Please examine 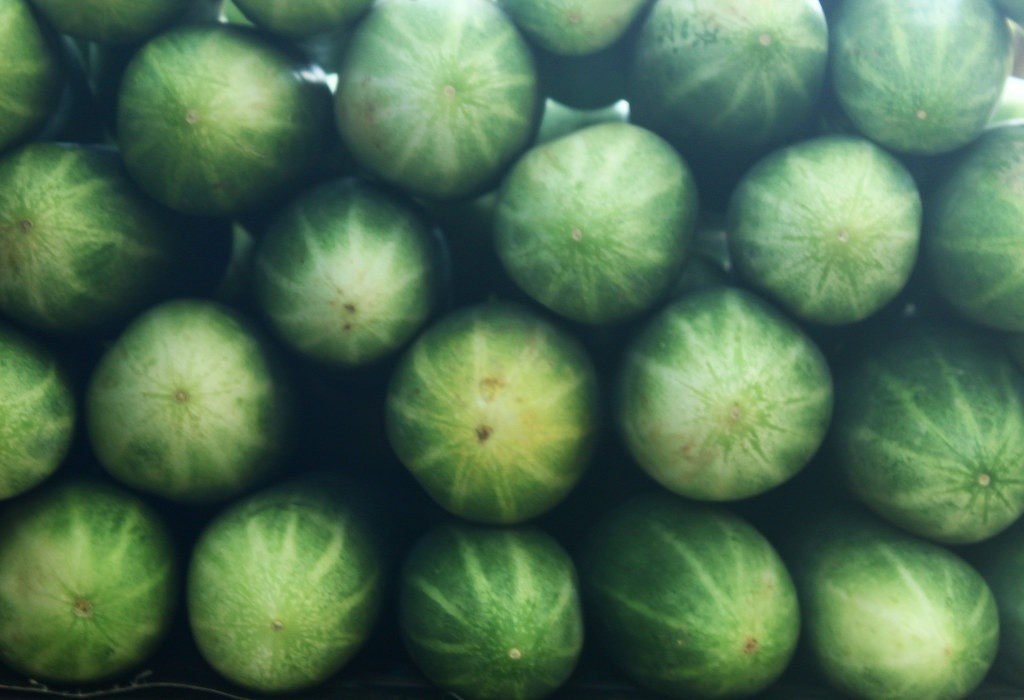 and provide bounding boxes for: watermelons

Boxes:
[0,0,1024,700]
